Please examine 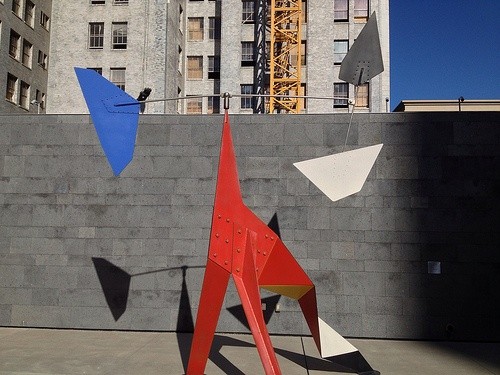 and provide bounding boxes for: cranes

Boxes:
[262,0,302,113]
